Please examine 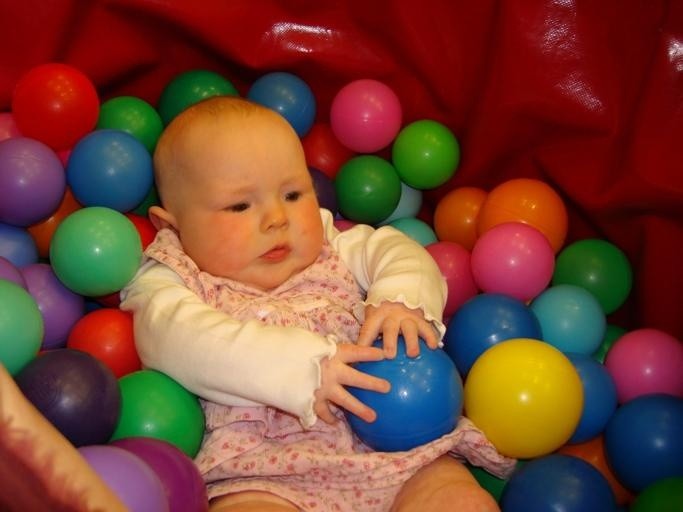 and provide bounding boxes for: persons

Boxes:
[120,96,502,512]
